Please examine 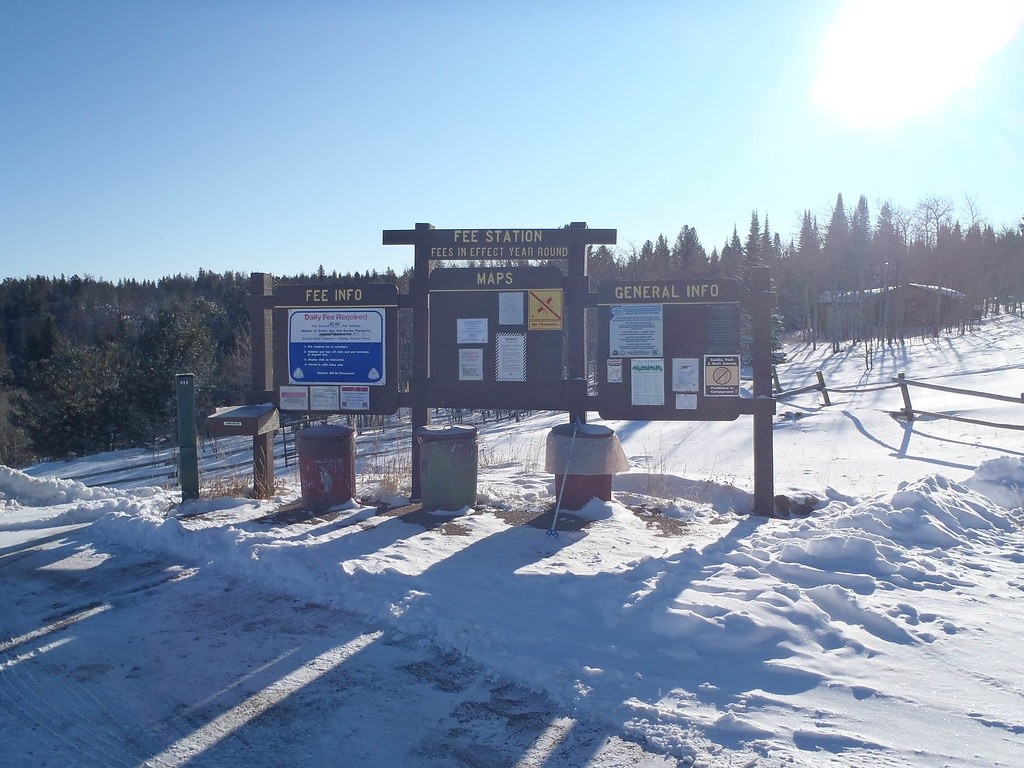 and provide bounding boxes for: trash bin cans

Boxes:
[414,423,478,515]
[294,420,356,512]
[551,423,616,510]
